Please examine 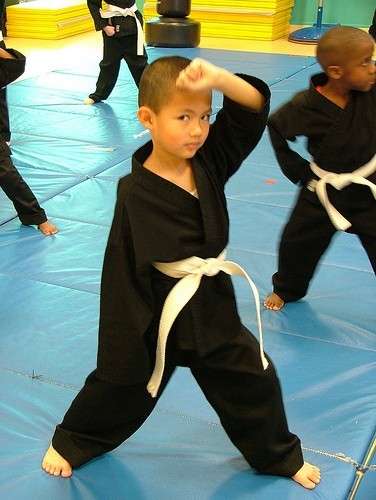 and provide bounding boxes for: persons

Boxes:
[263,26,376,310]
[84,0,149,104]
[41,56,321,490]
[0,0,58,236]
